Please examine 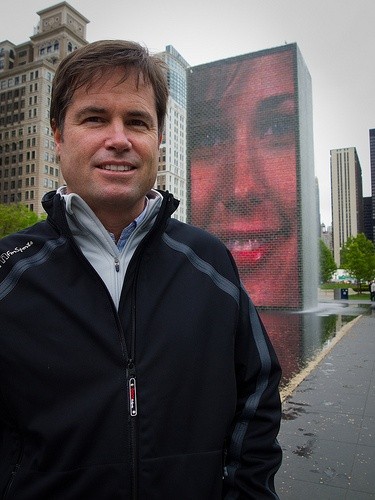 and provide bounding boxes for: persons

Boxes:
[0,40,282,500]
[369,280,375,301]
[187,52,299,309]
